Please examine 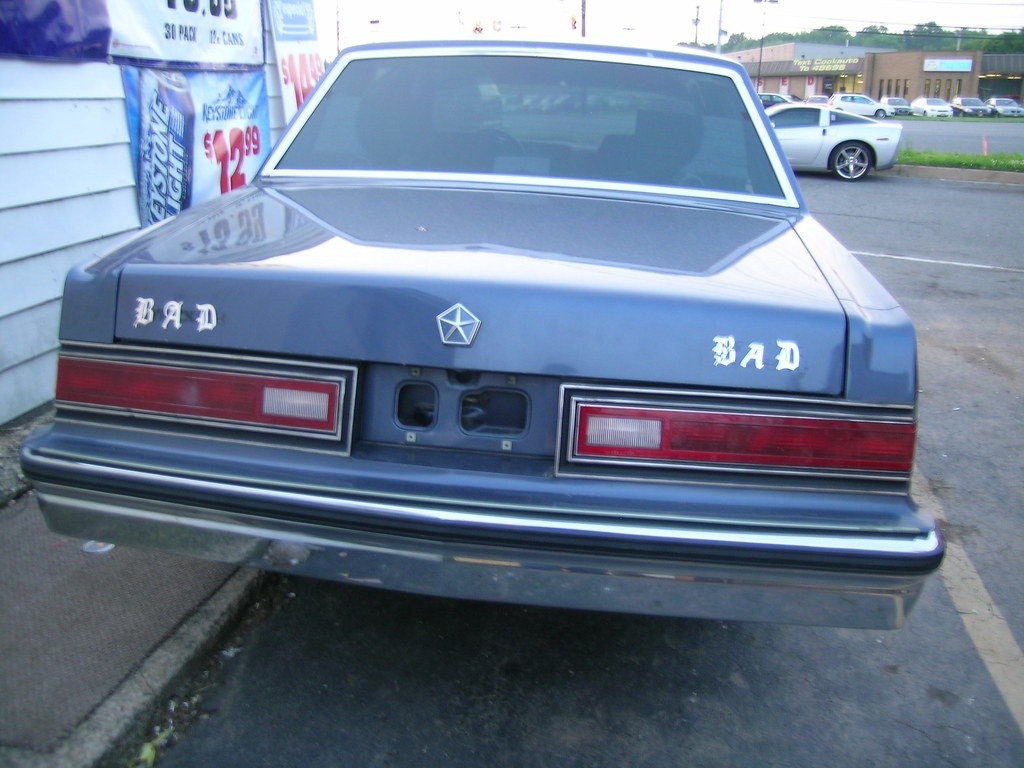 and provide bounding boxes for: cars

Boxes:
[762,101,905,184]
[948,95,993,120]
[984,97,1024,119]
[879,95,915,118]
[828,93,897,119]
[14,40,948,632]
[760,90,829,121]
[911,96,954,120]
